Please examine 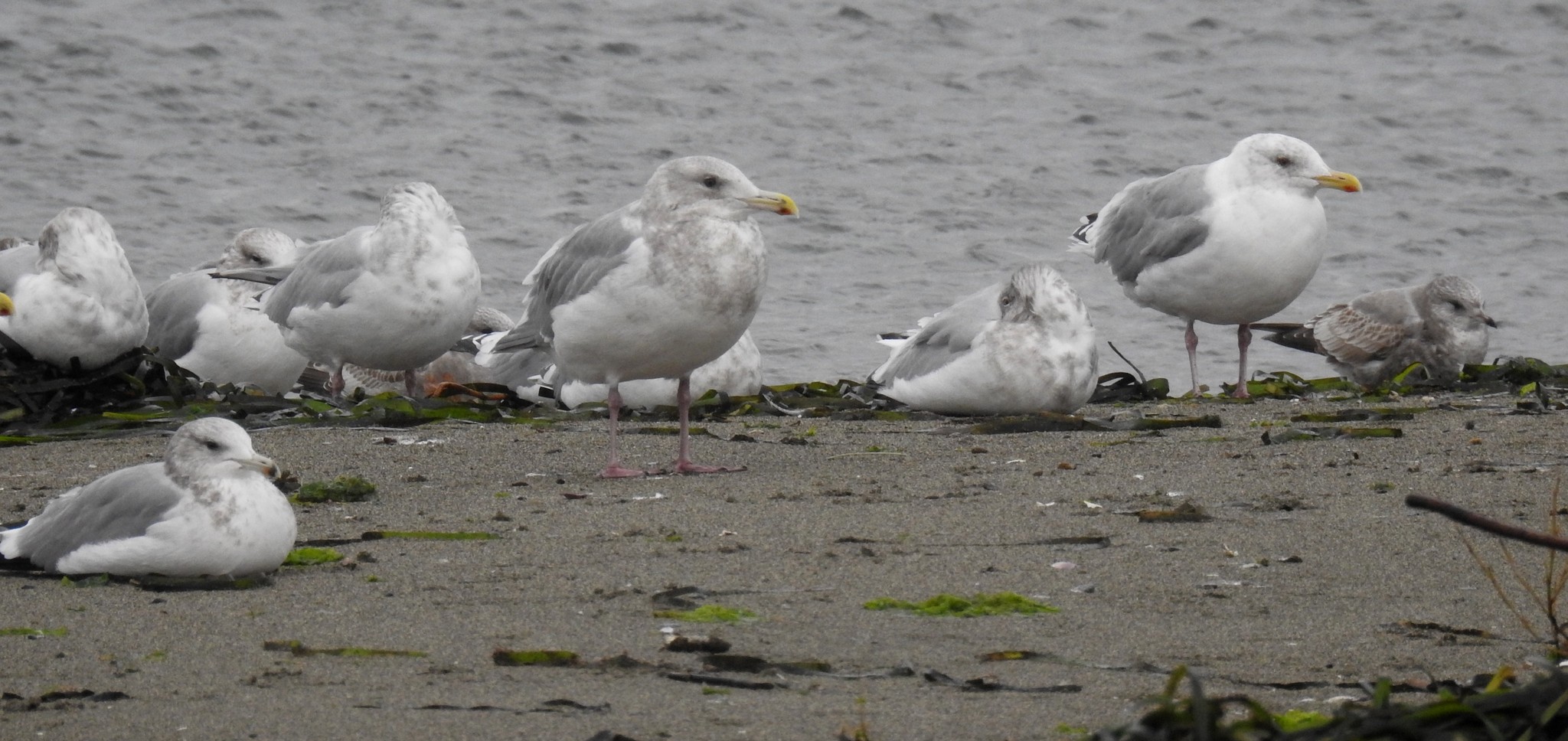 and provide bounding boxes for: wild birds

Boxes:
[850,261,1101,415]
[1064,132,1363,398]
[1251,275,1502,388]
[1,155,802,408]
[0,417,300,579]
